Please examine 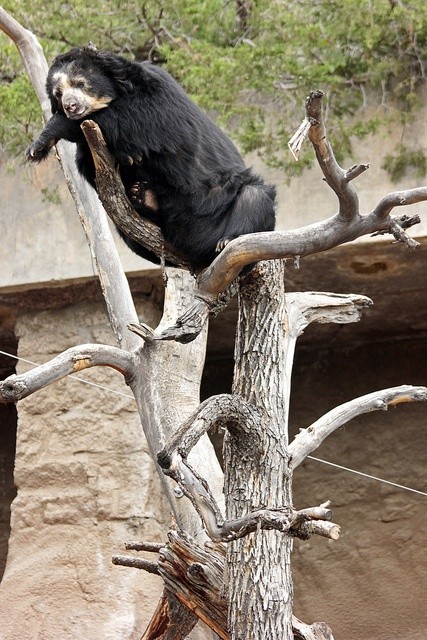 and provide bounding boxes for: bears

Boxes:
[25,46,281,275]
